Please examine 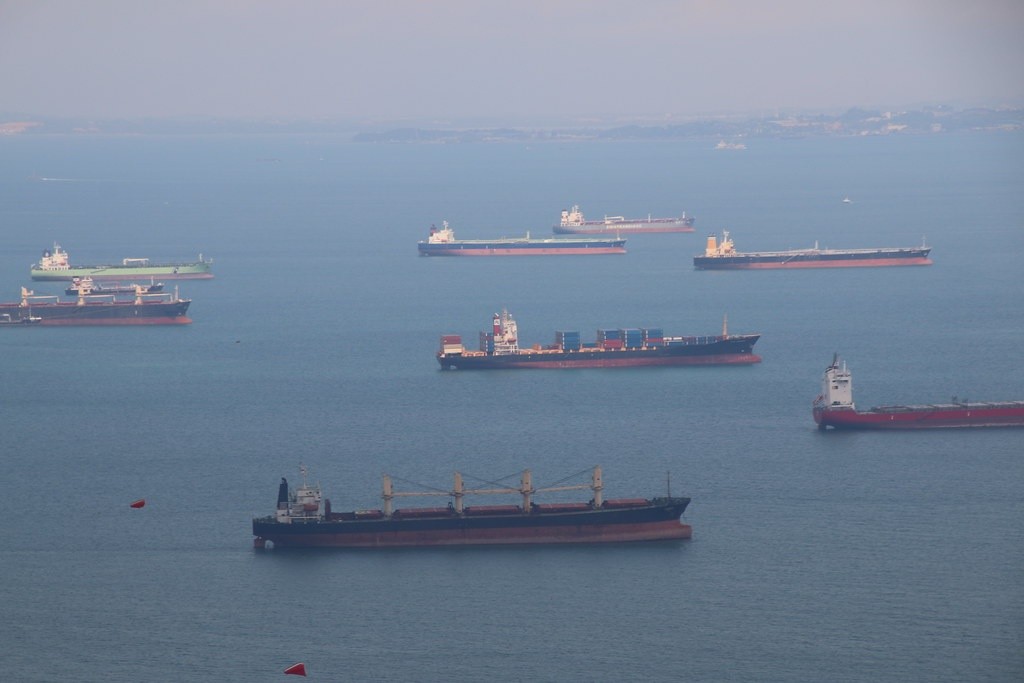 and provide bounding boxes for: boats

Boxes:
[812,360,1024,427]
[0,287,194,325]
[554,204,694,232]
[250,464,694,549]
[418,224,627,258]
[693,230,933,268]
[437,312,763,368]
[65,275,163,292]
[30,242,214,280]
